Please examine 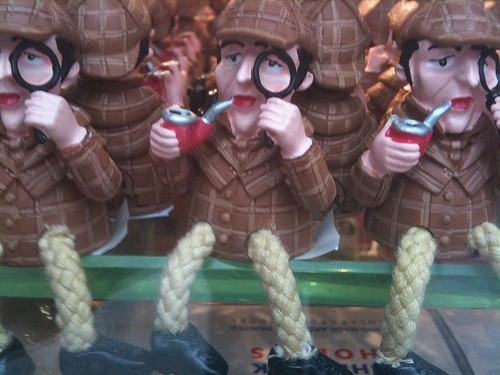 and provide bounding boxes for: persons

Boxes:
[346,0,500,375]
[299,1,419,195]
[0,0,155,375]
[150,0,355,375]
[79,1,202,213]
